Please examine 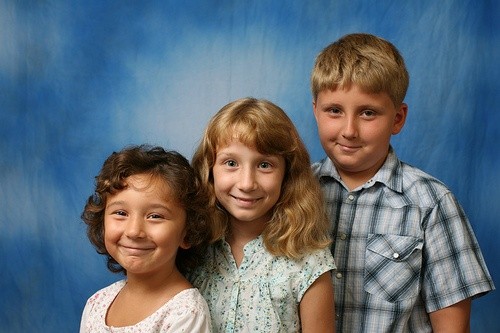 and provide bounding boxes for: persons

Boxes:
[79,144,216,333]
[308,33,496,333]
[185,97,338,333]
